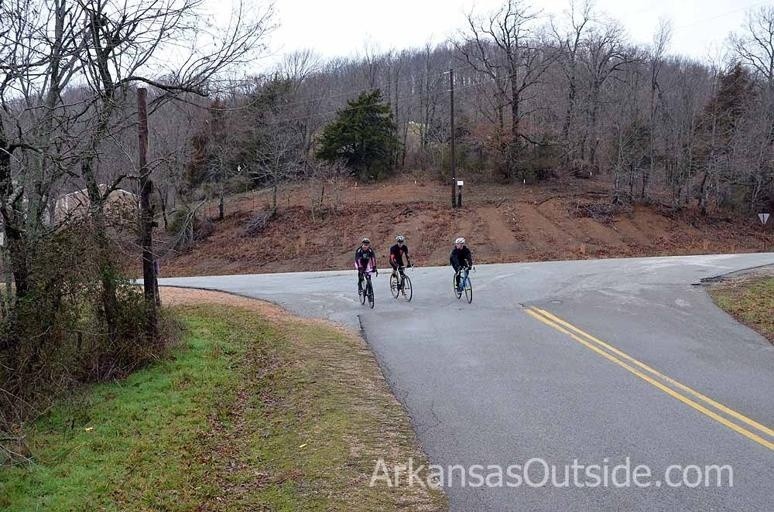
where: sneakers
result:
[457,287,462,293]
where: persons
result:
[388,236,412,291]
[355,238,376,303]
[450,238,473,291]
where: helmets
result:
[361,237,370,243]
[456,237,465,244]
[396,236,404,242]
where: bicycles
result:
[356,269,379,310]
[451,267,478,305]
[388,265,414,302]
